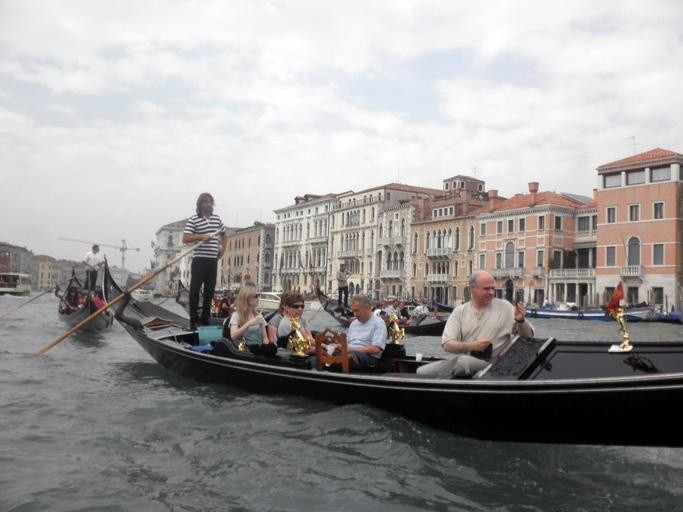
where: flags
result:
[604,281,623,317]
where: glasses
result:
[291,304,304,309]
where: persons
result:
[204,274,256,318]
[370,295,439,328]
[320,294,388,373]
[514,318,524,322]
[416,269,534,379]
[182,192,227,327]
[272,294,315,364]
[62,286,107,314]
[616,307,630,348]
[229,286,269,355]
[267,291,313,346]
[81,244,103,289]
[335,264,351,308]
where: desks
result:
[377,352,447,375]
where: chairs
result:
[348,342,406,373]
[264,304,309,367]
[212,311,264,362]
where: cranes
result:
[52,236,142,279]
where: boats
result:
[313,277,449,338]
[57,264,113,333]
[255,290,311,312]
[0,272,33,297]
[99,253,682,449]
[517,299,656,324]
[131,287,152,302]
[172,279,272,329]
